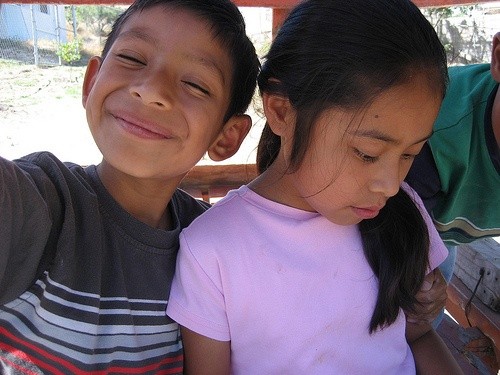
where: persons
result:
[165,0,466,375]
[0,1,241,374]
[404,31,500,330]
